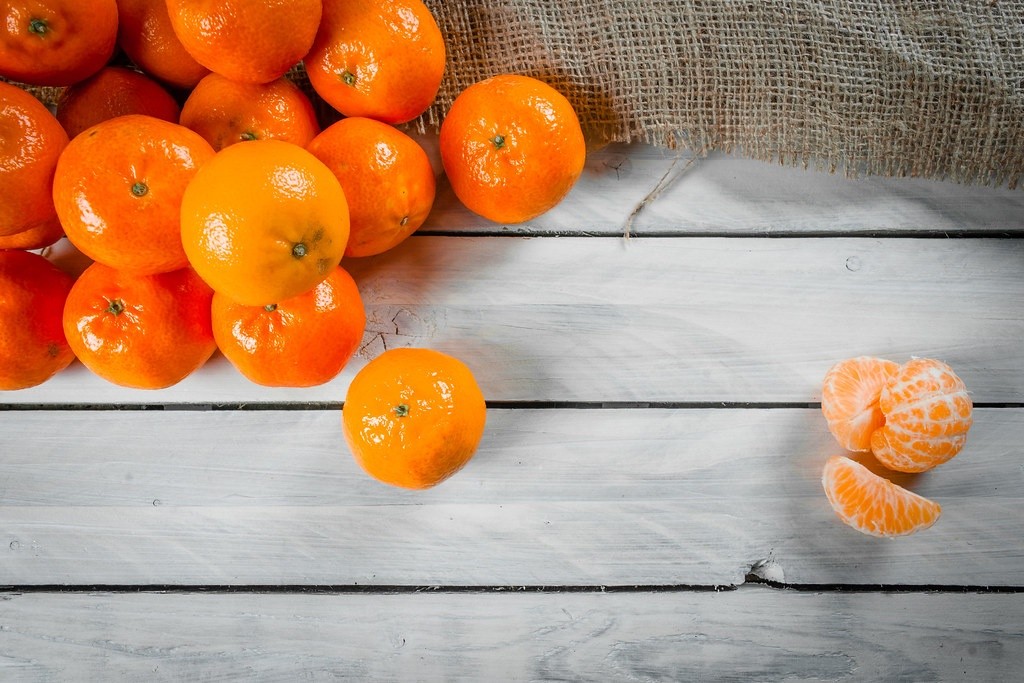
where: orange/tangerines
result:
[343,348,486,490]
[0,0,586,389]
[819,357,973,539]
